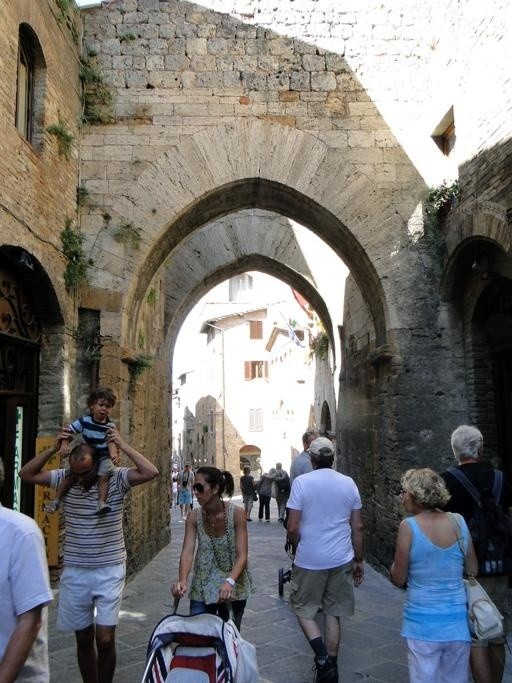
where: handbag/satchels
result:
[254,491,258,501]
[447,511,504,642]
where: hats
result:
[184,461,193,467]
[403,467,451,505]
[306,437,334,456]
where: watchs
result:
[226,577,236,587]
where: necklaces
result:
[205,501,233,572]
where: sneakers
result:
[95,503,112,516]
[44,499,61,514]
[314,655,338,683]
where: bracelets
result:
[352,557,364,563]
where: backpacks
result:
[445,466,510,578]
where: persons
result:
[240,466,256,521]
[286,436,365,683]
[171,467,255,638]
[43,387,120,515]
[261,463,289,523]
[0,457,54,683]
[18,423,160,683]
[440,424,512,683]
[390,467,479,683]
[176,464,193,521]
[289,432,318,490]
[256,468,275,523]
[171,465,178,509]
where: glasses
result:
[192,482,204,493]
[71,464,97,476]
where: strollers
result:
[140,597,257,682]
